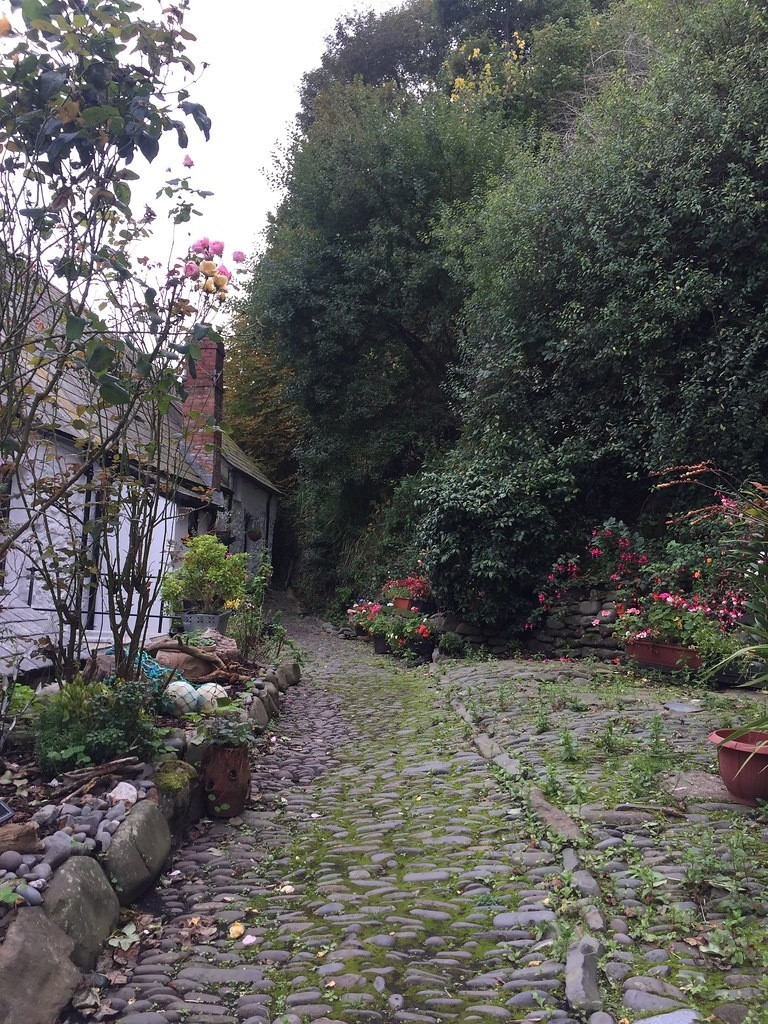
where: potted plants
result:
[159,534,253,636]
[181,702,261,818]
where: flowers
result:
[383,570,430,602]
[607,605,727,646]
[369,602,403,644]
[345,598,373,624]
[403,605,436,646]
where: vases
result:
[354,624,369,636]
[413,640,434,655]
[393,597,412,611]
[624,638,704,671]
[707,728,768,807]
[372,632,390,654]
